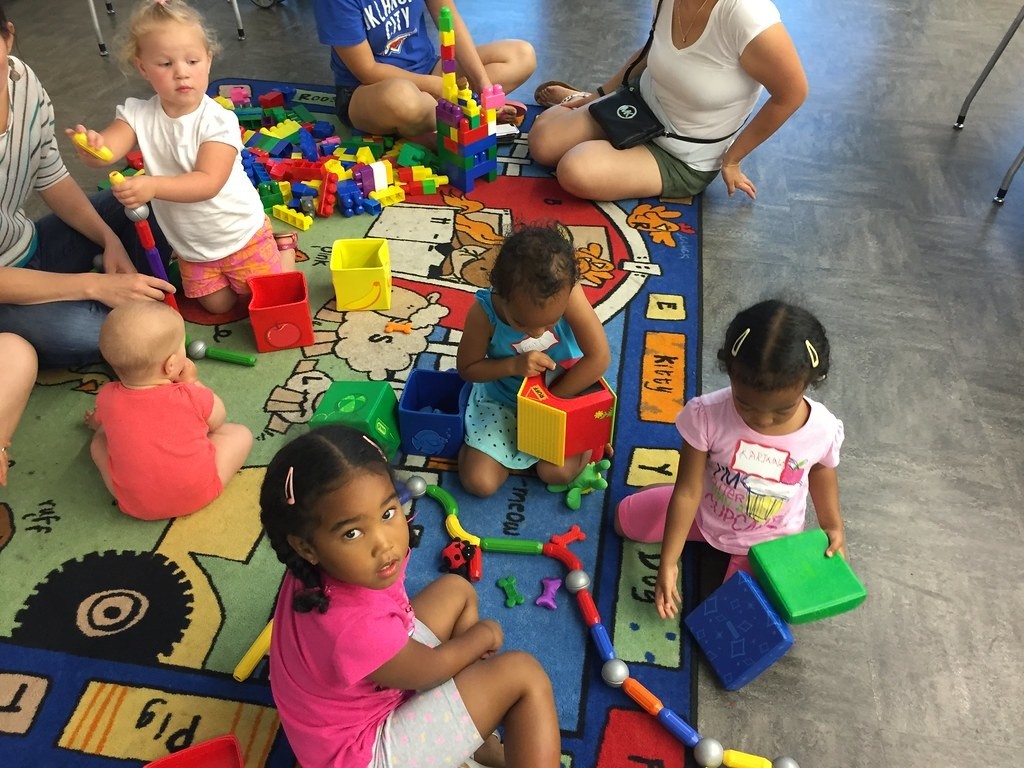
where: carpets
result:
[0,77,711,768]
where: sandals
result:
[272,229,300,250]
[496,101,529,124]
[534,78,595,108]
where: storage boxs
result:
[247,271,315,354]
[517,356,617,466]
[308,382,401,461]
[329,238,391,311]
[397,367,471,458]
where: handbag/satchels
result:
[588,83,669,148]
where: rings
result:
[2,447,7,453]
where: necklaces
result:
[677,0,708,42]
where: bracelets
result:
[596,87,605,97]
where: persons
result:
[614,299,846,619]
[84,301,253,521]
[0,332,39,487]
[456,226,611,498]
[528,0,808,202]
[65,0,299,314]
[311,0,536,140]
[0,8,176,376]
[259,425,560,768]
